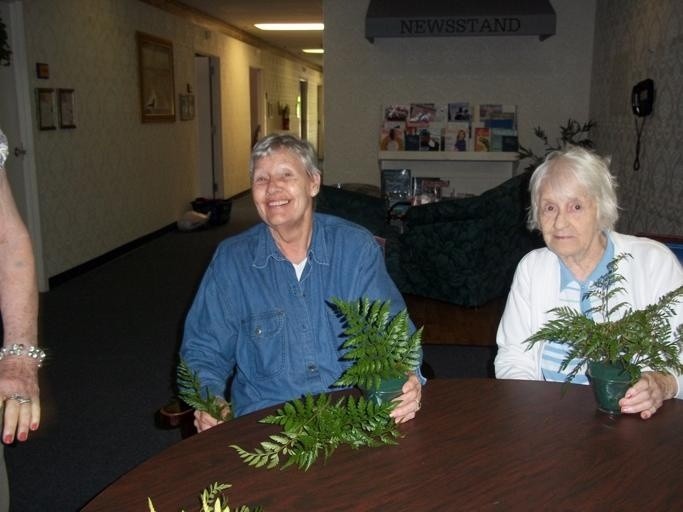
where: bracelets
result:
[0,341,49,369]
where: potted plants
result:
[524,248,683,419]
[326,297,420,416]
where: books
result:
[380,168,448,220]
[379,99,522,153]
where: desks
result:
[64,377,683,510]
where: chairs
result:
[402,173,537,311]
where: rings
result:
[413,398,423,412]
[8,392,24,401]
[18,397,33,405]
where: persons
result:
[176,132,428,434]
[493,144,682,420]
[0,129,44,511]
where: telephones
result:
[631,78,654,117]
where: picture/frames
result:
[136,30,177,125]
